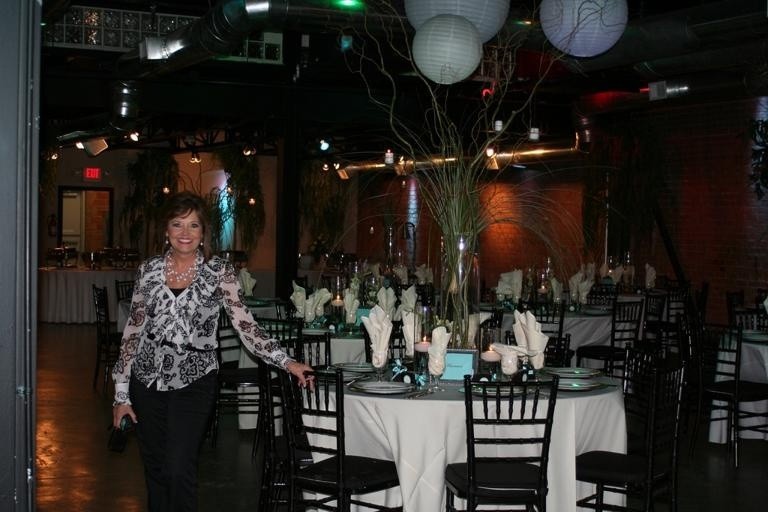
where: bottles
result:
[329,245,637,395]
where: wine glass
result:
[291,305,326,330]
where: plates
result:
[299,326,603,400]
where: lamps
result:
[45,131,258,163]
[323,144,499,171]
[405,0,629,84]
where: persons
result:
[111,189,315,511]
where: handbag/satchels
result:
[106,413,134,454]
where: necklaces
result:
[163,246,204,283]
